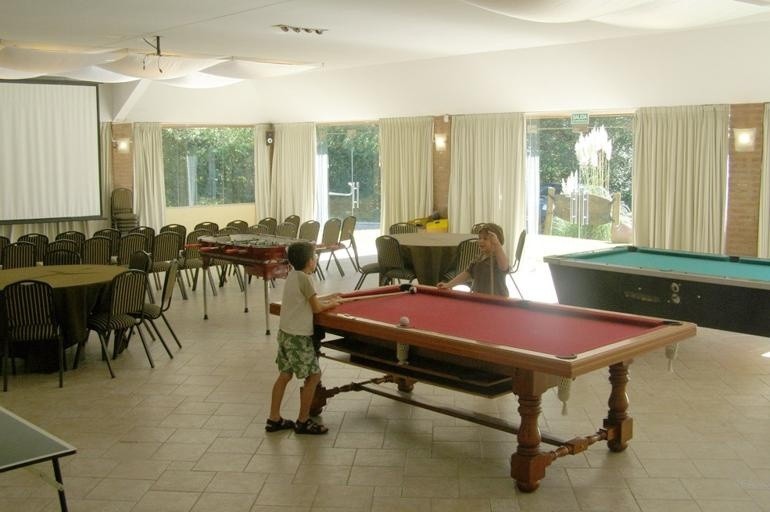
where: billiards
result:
[408,286,418,295]
[399,315,410,326]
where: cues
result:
[490,233,495,297]
[334,290,410,301]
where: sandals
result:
[295,419,328,434]
[266,417,293,431]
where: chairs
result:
[2,280,70,391]
[105,187,142,238]
[126,259,183,361]
[73,269,157,378]
[348,218,528,301]
[122,249,153,349]
[1,213,361,296]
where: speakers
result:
[266,132,273,145]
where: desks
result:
[195,232,315,336]
[541,244,770,374]
[268,284,698,495]
[0,403,78,511]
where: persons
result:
[265,241,343,435]
[436,222,510,298]
